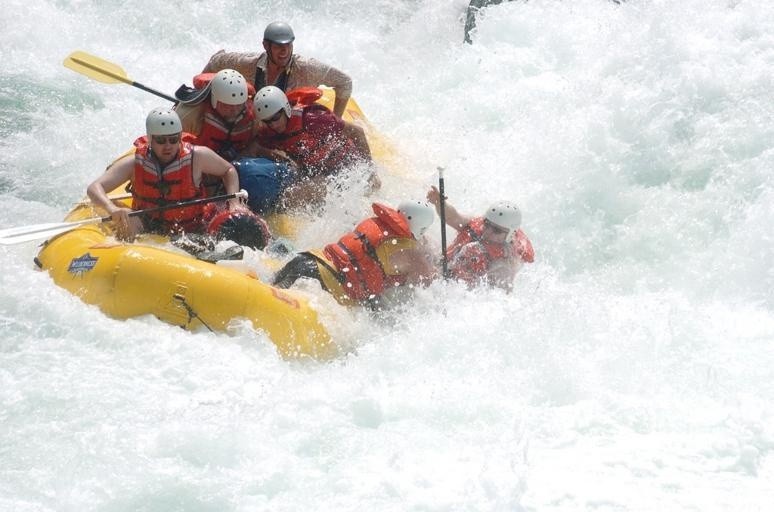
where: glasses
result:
[153,135,178,144]
[484,221,506,234]
[262,109,283,124]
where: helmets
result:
[397,196,435,240]
[146,106,182,141]
[264,21,295,44]
[211,69,248,108]
[484,200,521,243]
[254,86,291,123]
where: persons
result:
[263,186,535,304]
[87,21,381,262]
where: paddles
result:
[0,188,248,245]
[62,50,178,104]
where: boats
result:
[32,89,404,368]
